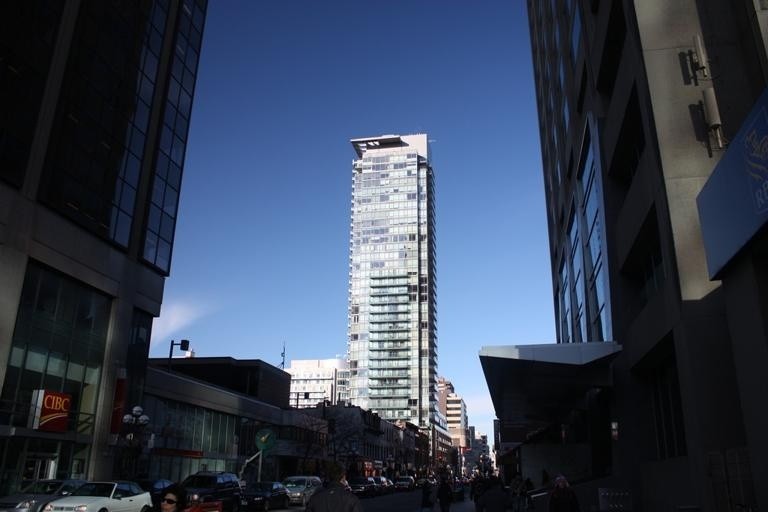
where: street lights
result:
[122,406,150,484]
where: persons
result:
[470,466,580,511]
[435,474,456,512]
[305,462,362,512]
[421,481,435,512]
[160,485,188,512]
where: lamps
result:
[688,33,732,159]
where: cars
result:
[0,479,92,512]
[43,479,154,512]
[181,471,243,512]
[351,476,434,498]
[284,475,324,506]
[242,481,291,510]
